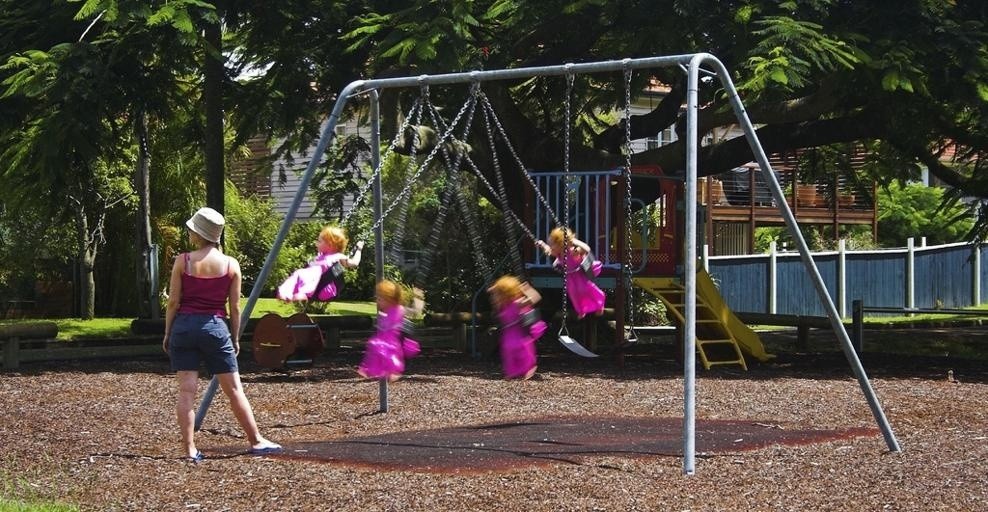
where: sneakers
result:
[251,439,282,452]
[185,450,210,464]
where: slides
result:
[697,268,767,362]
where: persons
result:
[161,207,281,461]
[531,226,606,322]
[486,275,549,382]
[354,279,423,384]
[275,227,365,305]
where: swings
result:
[558,70,640,357]
[307,85,594,346]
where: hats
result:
[186,207,225,244]
